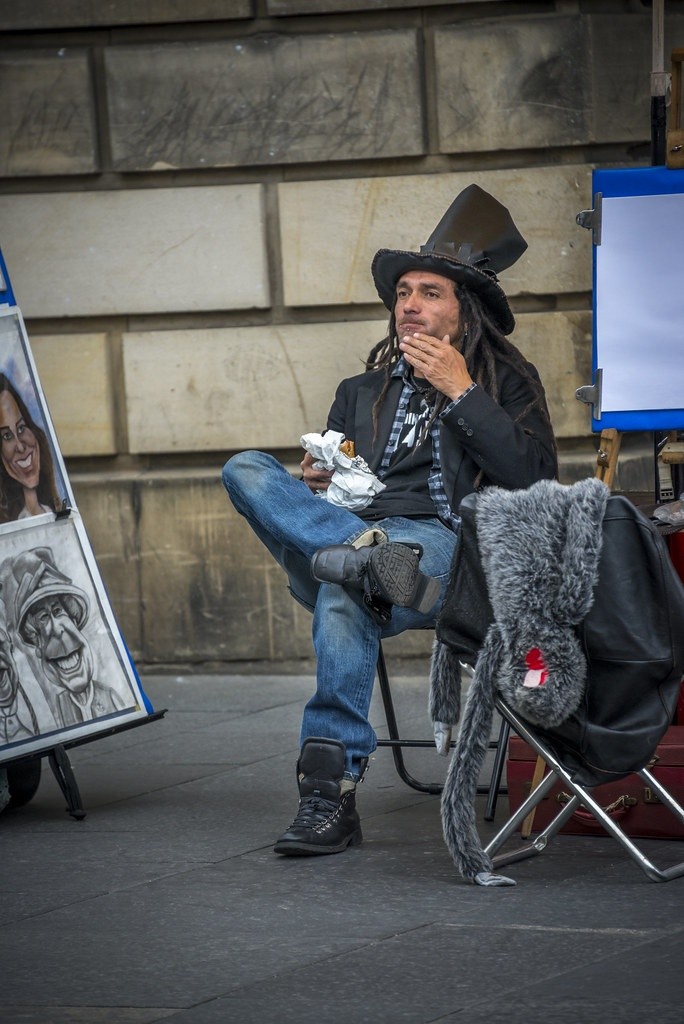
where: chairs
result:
[365,493,684,882]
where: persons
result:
[221,185,561,856]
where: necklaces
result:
[410,371,434,394]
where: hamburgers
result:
[340,439,354,460]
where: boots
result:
[311,541,443,614]
[272,738,369,853]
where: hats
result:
[371,183,527,337]
